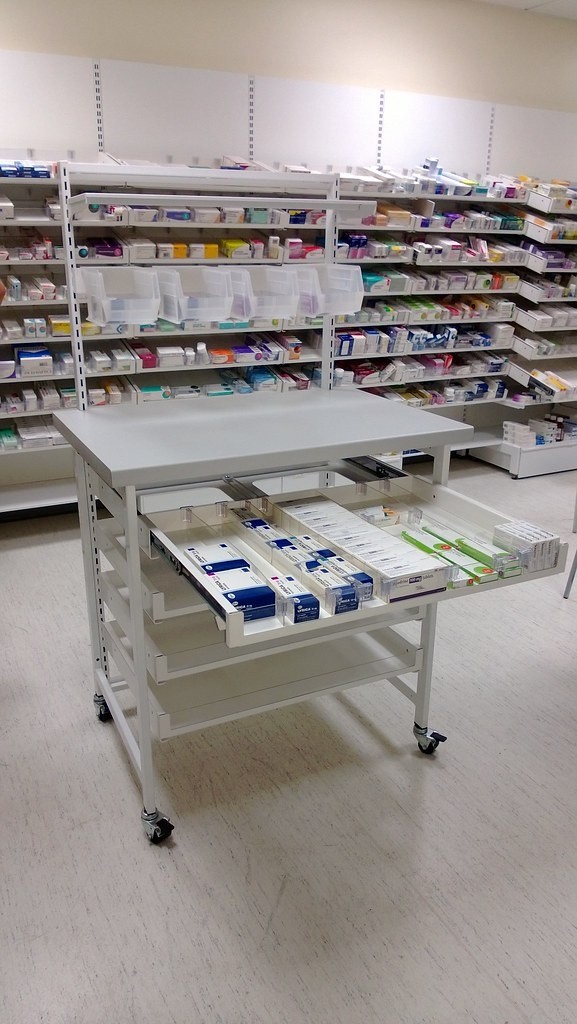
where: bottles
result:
[544,413,565,441]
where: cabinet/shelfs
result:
[466,190,577,481]
[0,176,529,514]
[50,162,573,847]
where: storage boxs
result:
[0,157,577,626]
